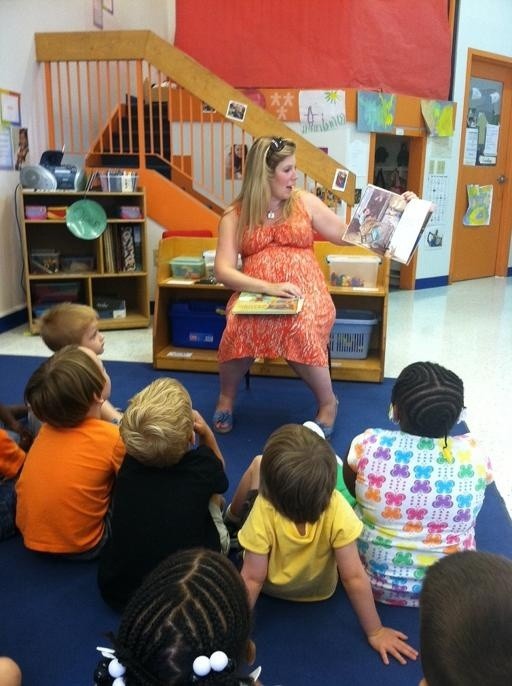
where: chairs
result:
[162,229,213,240]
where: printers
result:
[39,150,80,192]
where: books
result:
[230,290,305,314]
[340,182,436,267]
[103,223,137,274]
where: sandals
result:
[211,405,234,434]
[313,395,340,441]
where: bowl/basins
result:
[99,175,138,192]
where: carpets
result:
[1,352,512,684]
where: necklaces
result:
[261,201,281,220]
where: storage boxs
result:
[166,299,230,351]
[31,302,74,324]
[324,254,382,288]
[203,249,243,282]
[31,280,82,304]
[28,250,63,274]
[166,255,205,280]
[62,254,96,270]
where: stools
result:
[225,291,337,394]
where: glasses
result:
[266,136,286,159]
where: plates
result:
[64,198,108,240]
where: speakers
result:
[20,163,57,190]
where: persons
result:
[40,301,127,424]
[95,376,231,614]
[13,128,30,171]
[94,544,265,684]
[224,422,419,667]
[342,362,494,610]
[0,654,23,685]
[211,134,421,443]
[14,342,129,566]
[1,404,33,547]
[418,550,511,685]
[226,146,248,176]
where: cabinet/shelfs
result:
[21,187,150,333]
[150,236,392,384]
